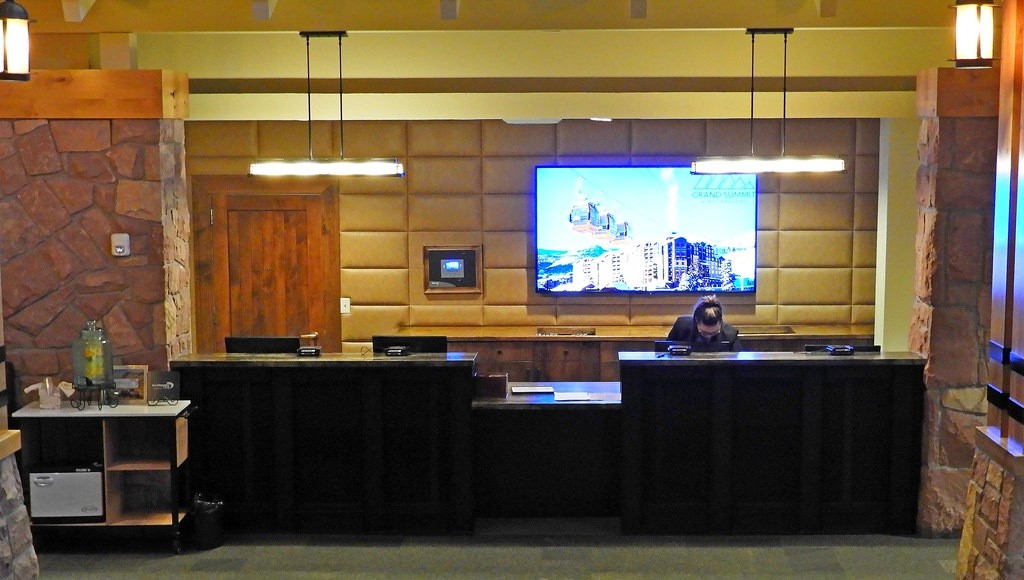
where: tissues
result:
[24,381,76,409]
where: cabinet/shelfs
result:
[11,400,199,555]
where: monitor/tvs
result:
[534,164,758,293]
[372,335,448,354]
[224,337,301,354]
[805,345,881,353]
[655,340,730,353]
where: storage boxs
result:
[28,472,104,524]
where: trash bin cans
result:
[190,492,229,550]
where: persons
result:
[666,294,743,352]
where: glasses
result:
[697,326,721,335]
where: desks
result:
[619,352,929,537]
[168,351,480,538]
[471,382,623,519]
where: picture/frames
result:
[101,364,149,406]
[422,245,484,295]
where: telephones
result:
[827,344,855,355]
[297,346,321,357]
[384,346,411,355]
[668,345,692,355]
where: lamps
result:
[0,0,40,82]
[689,28,846,175]
[247,31,404,178]
[946,0,1002,70]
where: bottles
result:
[71,328,112,388]
